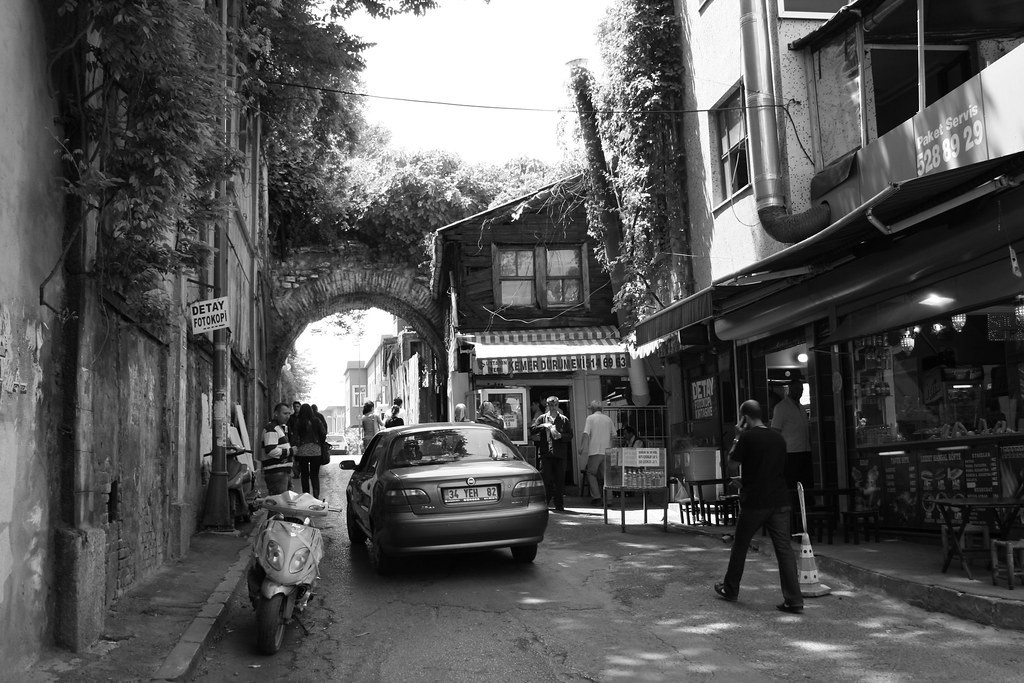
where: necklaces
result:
[757,425,766,427]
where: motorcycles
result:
[247,489,344,656]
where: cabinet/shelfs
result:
[603,447,668,533]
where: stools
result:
[719,496,739,518]
[790,511,836,545]
[704,500,728,526]
[990,536,1024,590]
[677,497,699,525]
[941,525,990,564]
[841,510,880,546]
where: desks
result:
[804,487,863,545]
[685,478,739,527]
[923,497,1024,580]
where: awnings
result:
[631,265,811,394]
[454,325,634,377]
[711,151,1024,293]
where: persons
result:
[714,400,804,613]
[451,393,563,465]
[361,396,408,456]
[620,426,646,449]
[529,396,573,512]
[286,401,327,499]
[770,380,815,534]
[260,403,298,520]
[579,400,617,506]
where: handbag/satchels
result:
[321,441,331,465]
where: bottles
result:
[623,469,665,488]
[854,333,896,444]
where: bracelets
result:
[732,439,740,445]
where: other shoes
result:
[592,498,612,507]
[293,473,299,479]
[554,505,564,512]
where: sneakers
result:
[714,581,739,603]
[777,601,804,612]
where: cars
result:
[324,434,349,454]
[338,421,550,572]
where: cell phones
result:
[740,418,746,428]
